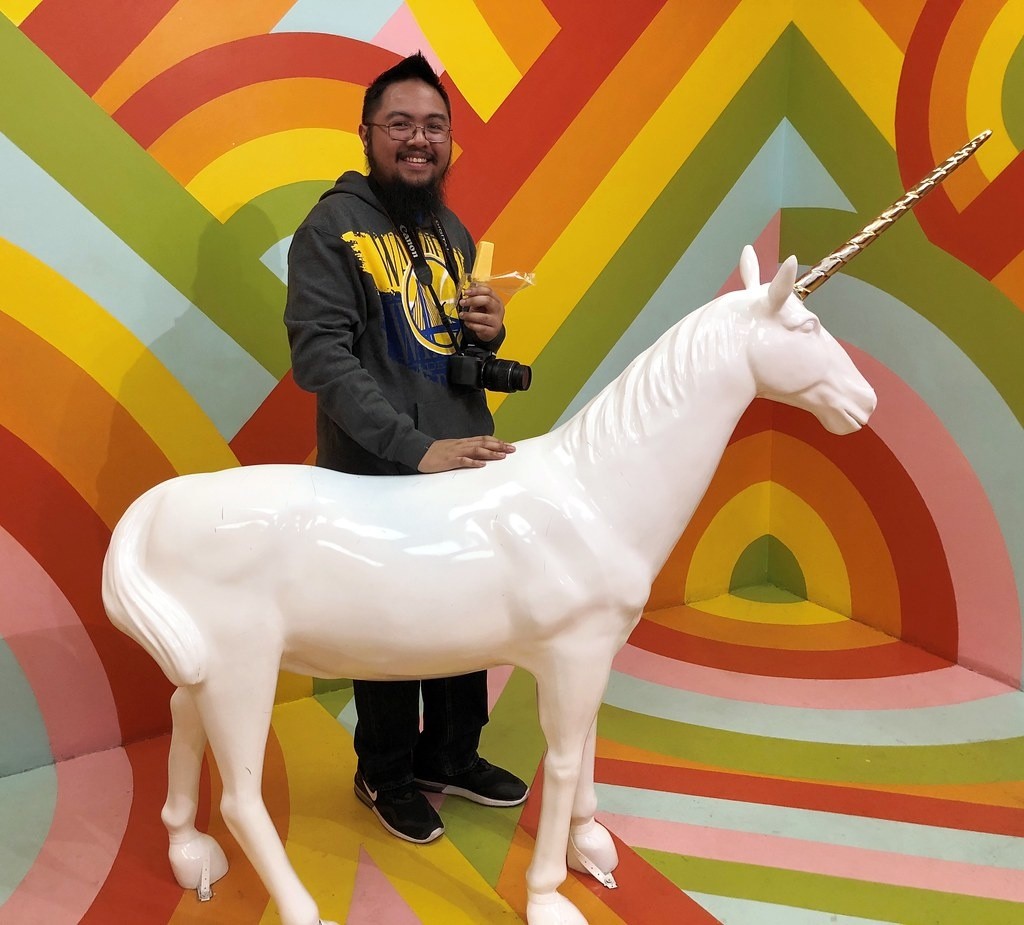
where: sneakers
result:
[411,751,531,807]
[354,769,444,844]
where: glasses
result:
[362,119,453,143]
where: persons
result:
[283,49,531,845]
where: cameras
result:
[450,346,532,393]
